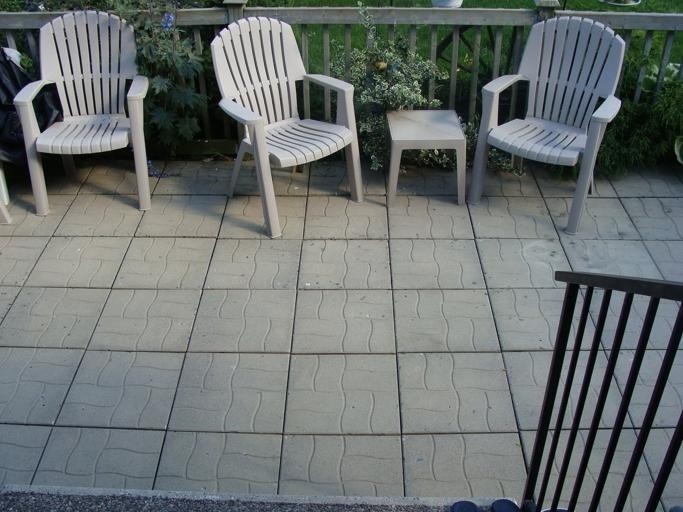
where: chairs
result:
[464,15,626,236]
[12,10,151,217]
[209,16,364,239]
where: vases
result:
[429,1,464,10]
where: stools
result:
[383,109,467,209]
